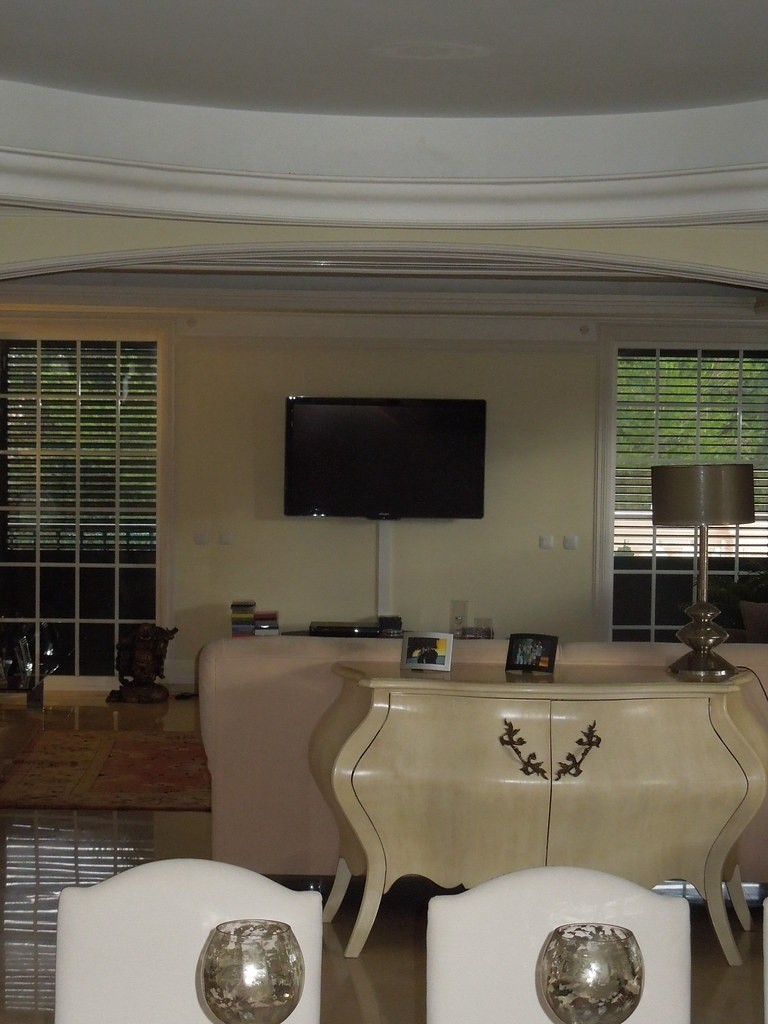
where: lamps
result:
[652,464,755,682]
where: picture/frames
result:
[400,632,453,672]
[504,633,558,674]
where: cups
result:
[450,600,468,638]
[474,618,492,635]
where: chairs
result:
[425,865,691,1024]
[54,857,322,1024]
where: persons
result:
[516,640,543,666]
[417,646,438,664]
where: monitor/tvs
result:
[284,396,486,518]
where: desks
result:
[308,660,768,966]
